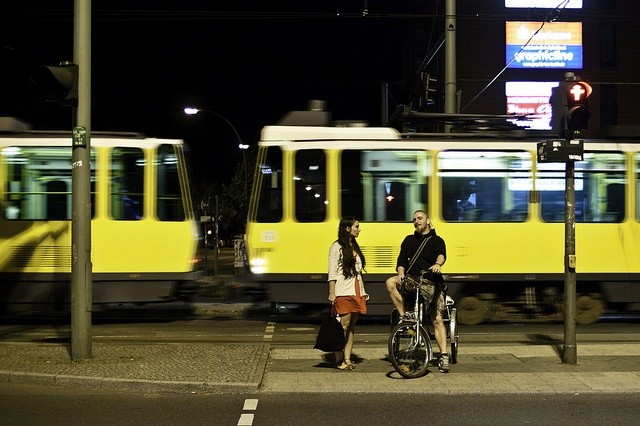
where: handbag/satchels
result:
[313,317,345,352]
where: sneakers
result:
[396,316,409,331]
[438,353,449,372]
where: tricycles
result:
[389,270,459,378]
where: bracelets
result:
[436,263,442,266]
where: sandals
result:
[333,360,352,369]
[344,359,356,369]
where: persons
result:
[328,217,369,369]
[386,211,449,372]
[457,193,475,220]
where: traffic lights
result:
[563,71,599,132]
[419,70,440,110]
[40,61,82,105]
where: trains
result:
[0,100,640,326]
